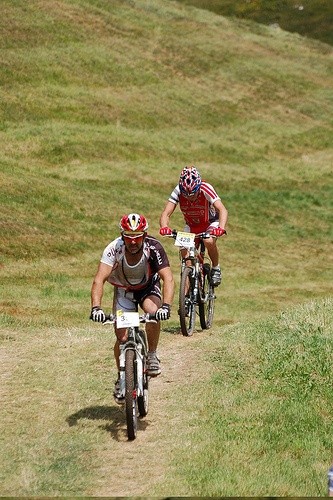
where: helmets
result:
[120,213,149,237]
[179,166,201,198]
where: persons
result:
[159,168,228,314]
[90,213,174,404]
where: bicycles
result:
[162,229,228,337]
[89,302,170,441]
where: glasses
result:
[123,234,143,243]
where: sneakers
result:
[210,264,222,286]
[178,297,189,314]
[114,379,126,405]
[145,352,162,376]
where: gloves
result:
[155,303,170,320]
[212,228,227,237]
[160,226,172,236]
[90,306,106,322]
